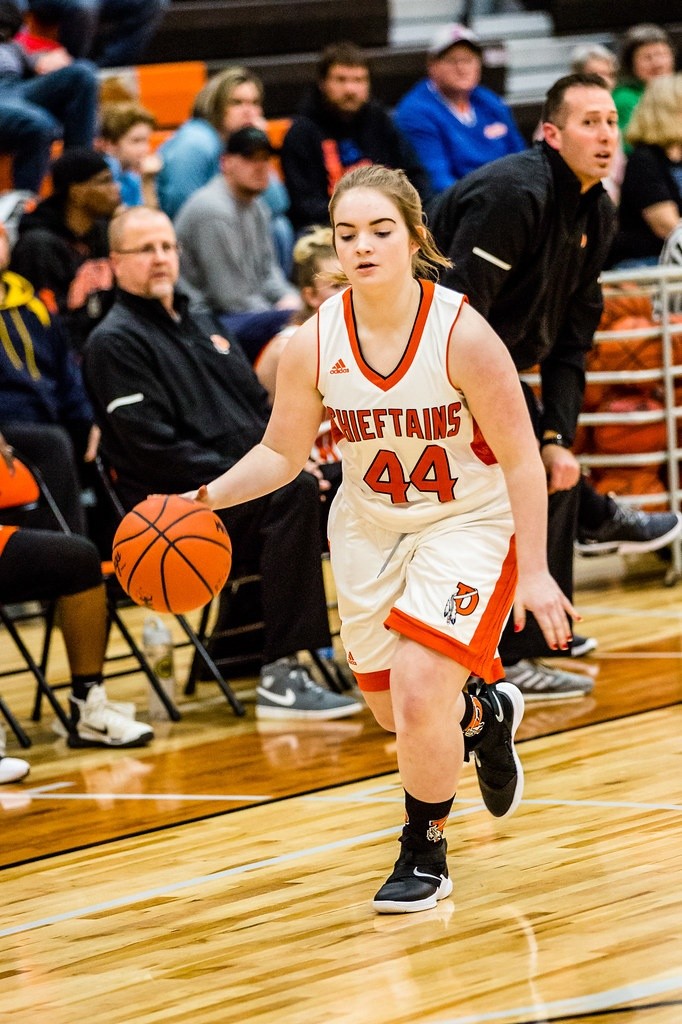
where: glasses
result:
[112,239,180,255]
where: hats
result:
[428,22,484,55]
[50,149,112,183]
[225,127,270,156]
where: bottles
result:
[141,614,175,716]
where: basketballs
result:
[111,495,233,614]
[519,281,682,513]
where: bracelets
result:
[541,439,571,449]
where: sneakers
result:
[67,682,155,749]
[0,754,31,784]
[503,661,593,700]
[574,491,682,560]
[255,659,362,719]
[570,635,597,657]
[372,826,453,912]
[467,682,525,821]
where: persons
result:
[148,164,584,915]
[0,0,682,788]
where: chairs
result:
[0,447,181,747]
[95,444,343,716]
